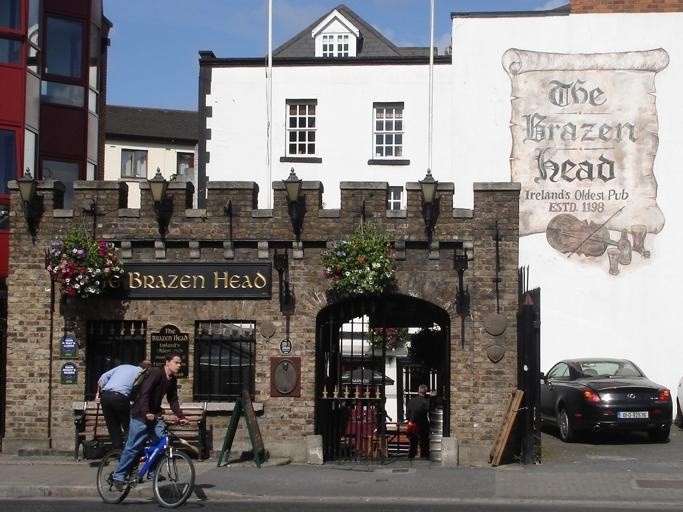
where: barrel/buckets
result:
[428,435,444,464]
[429,409,444,435]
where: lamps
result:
[16,167,42,223]
[281,166,304,225]
[418,167,438,229]
[146,166,170,223]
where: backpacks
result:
[132,366,163,390]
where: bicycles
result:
[95,416,195,508]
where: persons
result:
[374,396,392,434]
[406,384,431,458]
[108,351,189,488]
[94,360,152,461]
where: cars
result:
[540,358,672,440]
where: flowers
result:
[368,328,408,353]
[43,222,127,300]
[319,225,400,302]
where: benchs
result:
[73,402,207,463]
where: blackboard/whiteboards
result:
[489,389,523,458]
[241,387,267,454]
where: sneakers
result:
[147,471,166,482]
[107,473,124,492]
